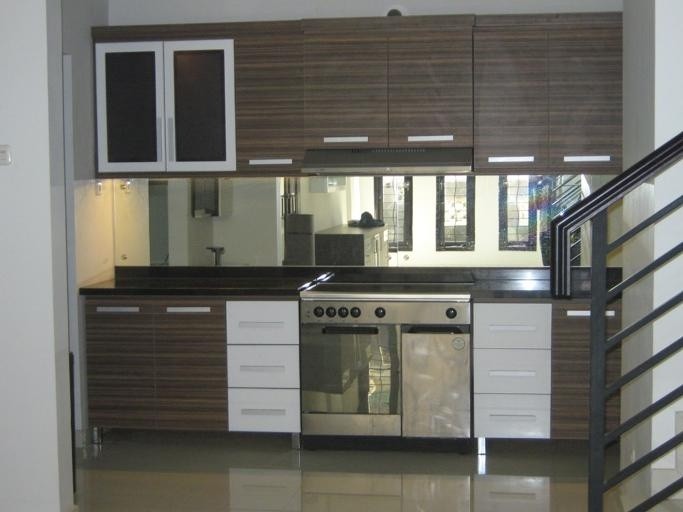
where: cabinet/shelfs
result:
[80,276,320,450]
[314,221,390,266]
[89,19,300,178]
[472,474,550,512]
[472,288,621,455]
[228,454,301,511]
[300,13,474,150]
[473,11,622,176]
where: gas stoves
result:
[300,283,470,325]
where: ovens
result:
[300,321,403,415]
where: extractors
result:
[299,148,475,176]
